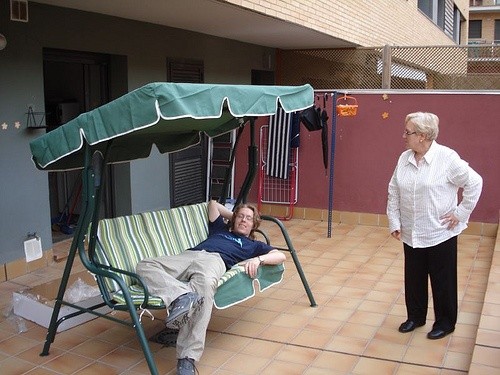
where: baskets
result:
[336,96,358,117]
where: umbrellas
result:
[321,93,329,176]
[300,95,322,132]
[290,112,300,147]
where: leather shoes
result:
[428,326,454,340]
[399,319,426,333]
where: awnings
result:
[29,84,314,172]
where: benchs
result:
[87,201,284,311]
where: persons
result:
[135,200,286,375]
[386,112,483,339]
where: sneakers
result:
[177,359,199,375]
[164,292,205,329]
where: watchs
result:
[258,256,264,265]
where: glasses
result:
[404,129,416,136]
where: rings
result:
[449,218,452,220]
